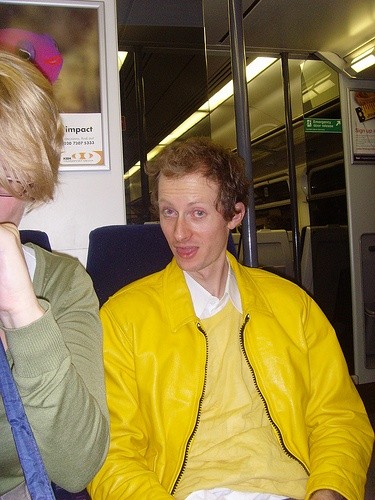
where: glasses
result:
[0,176,35,199]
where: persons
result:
[0,50,111,500]
[85,139,375,500]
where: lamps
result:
[350,46,375,74]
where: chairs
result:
[18,225,356,376]
